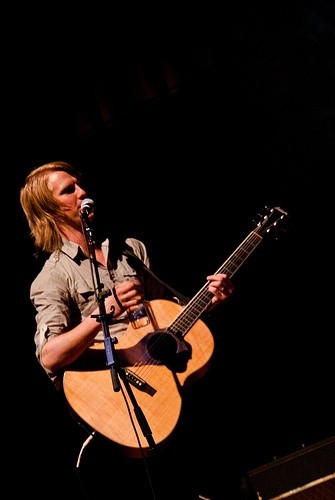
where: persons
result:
[20,162,235,384]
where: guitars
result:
[63,202,289,459]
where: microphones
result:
[78,198,94,216]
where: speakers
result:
[243,437,334,498]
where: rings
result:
[219,287,226,291]
